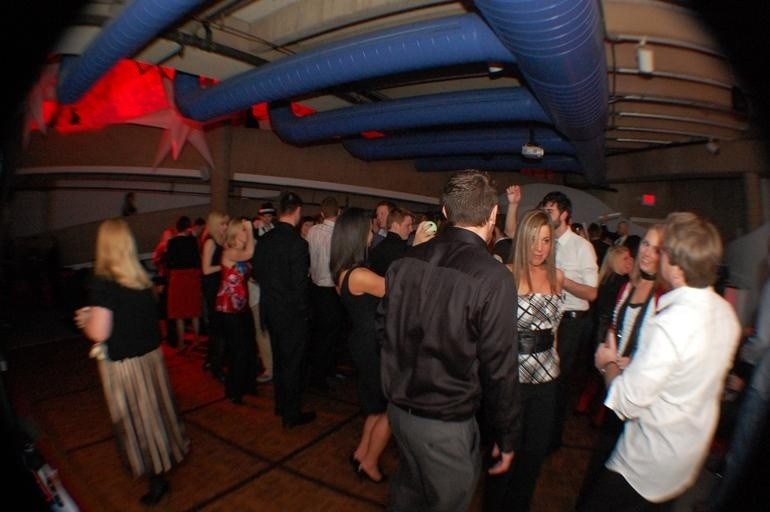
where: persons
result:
[153,169,769,512]
[71,216,195,509]
[120,190,138,216]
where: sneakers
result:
[274,404,317,430]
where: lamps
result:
[636,36,655,76]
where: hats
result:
[257,203,277,216]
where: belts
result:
[394,403,473,422]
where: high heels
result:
[349,453,390,484]
[139,481,170,505]
